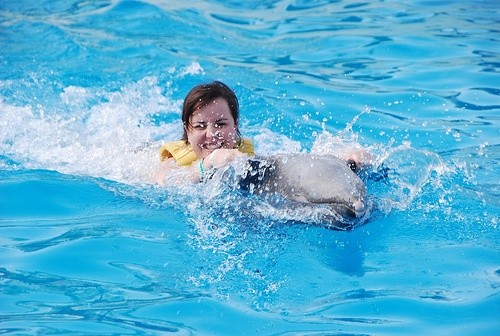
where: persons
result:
[160,79,255,185]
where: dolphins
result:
[255,154,366,231]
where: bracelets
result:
[197,157,207,180]
